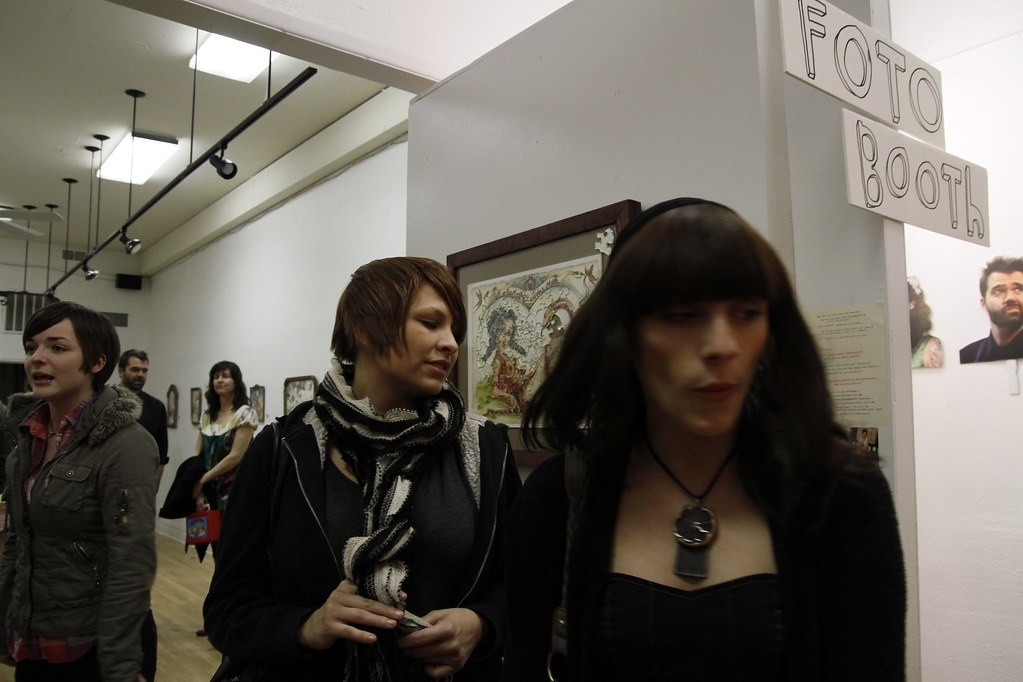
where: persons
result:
[480,196,906,682]
[201,256,523,682]
[185,360,258,651]
[959,256,1023,365]
[907,275,943,368]
[112,349,169,497]
[0,301,160,681]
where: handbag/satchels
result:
[186,503,222,544]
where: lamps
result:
[80,265,101,282]
[209,154,238,180]
[96,130,180,186]
[119,235,142,255]
[188,32,281,85]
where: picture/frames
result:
[248,384,265,425]
[165,383,179,430]
[283,376,319,417]
[905,253,1023,369]
[190,387,202,426]
[441,199,644,475]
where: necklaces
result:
[640,424,740,583]
